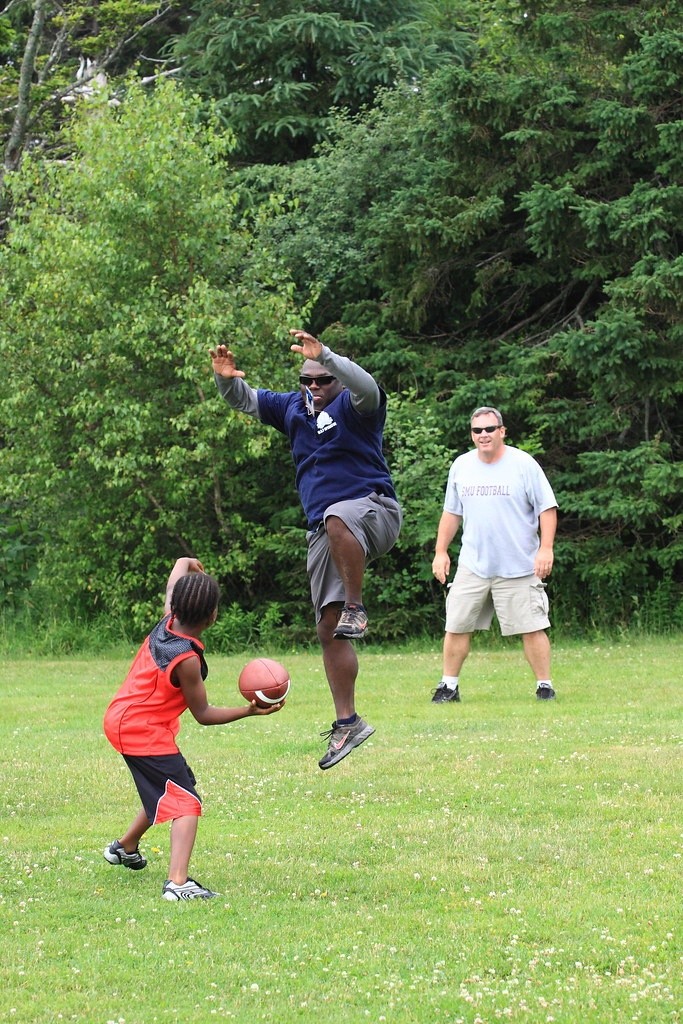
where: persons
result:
[208,327,403,770]
[101,556,286,903]
[430,407,558,705]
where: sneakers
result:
[330,602,371,639]
[432,681,461,704]
[315,712,375,768]
[102,840,147,870]
[535,683,556,702]
[160,878,224,903]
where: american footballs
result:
[238,658,291,709]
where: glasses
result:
[297,373,337,387]
[471,425,503,434]
